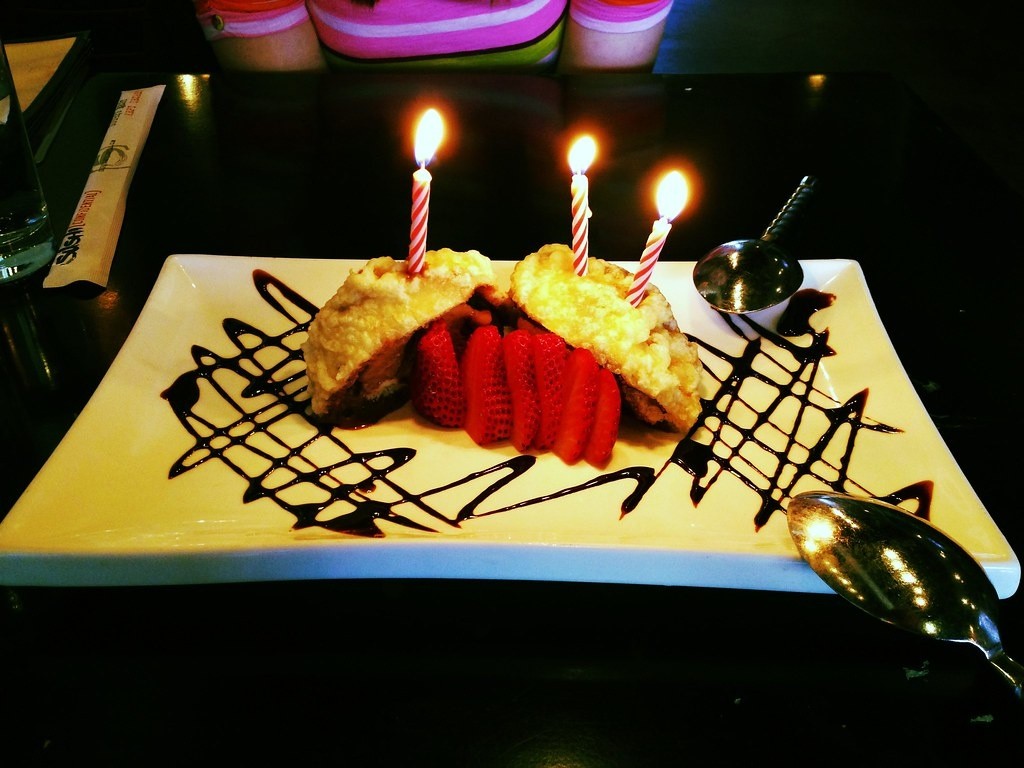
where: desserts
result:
[508,244,704,435]
[302,246,498,417]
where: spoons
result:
[787,490,1024,718]
[693,169,821,315]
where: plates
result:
[0,250,1020,614]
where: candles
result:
[406,107,446,275]
[564,133,599,277]
[626,168,693,308]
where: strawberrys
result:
[408,326,621,467]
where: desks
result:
[0,72,1024,768]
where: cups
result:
[0,38,55,287]
